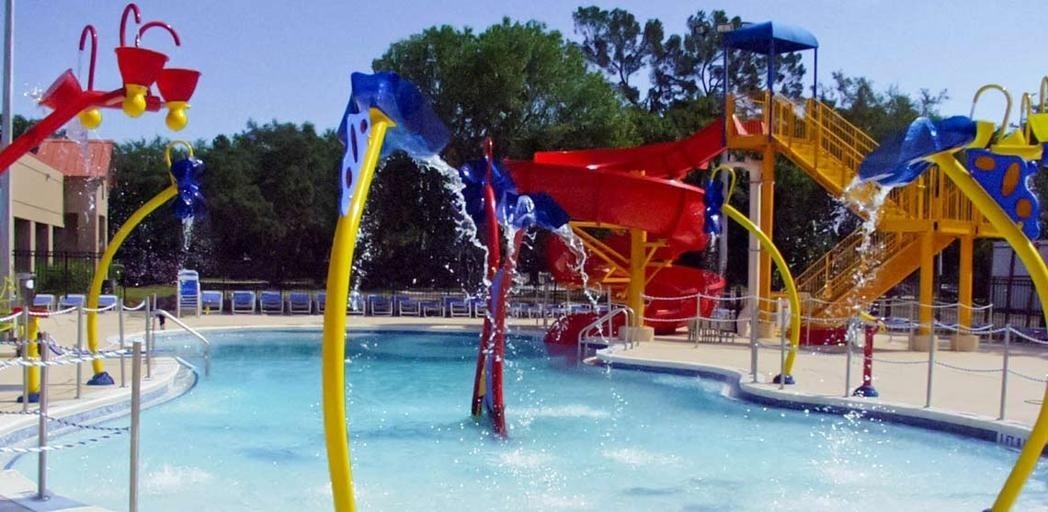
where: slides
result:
[504,116,769,344]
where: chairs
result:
[31,292,121,312]
[971,321,1048,346]
